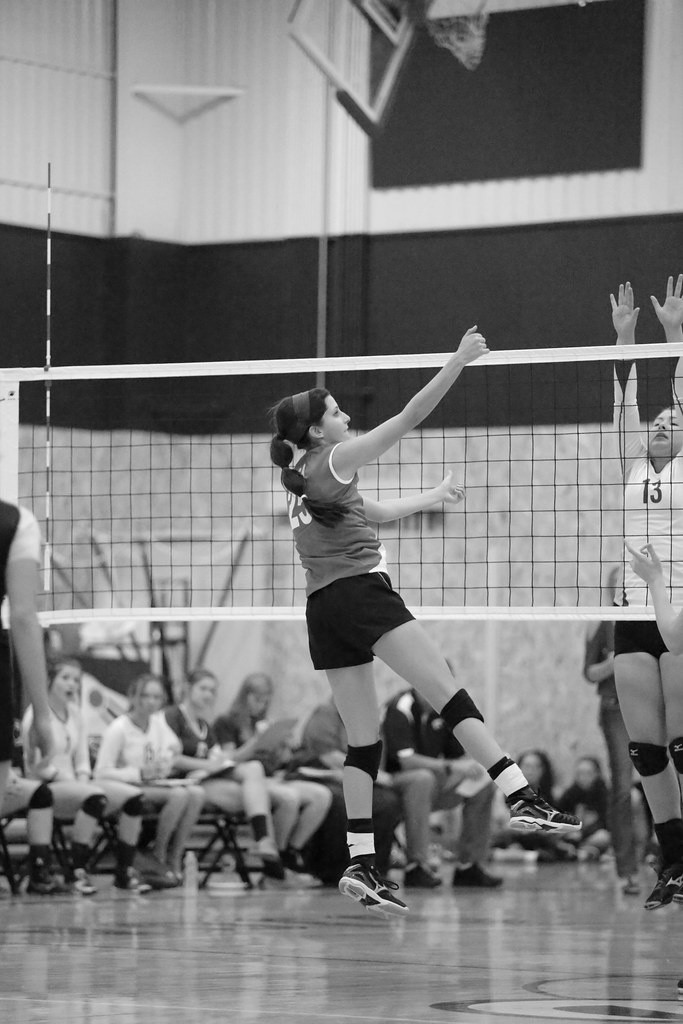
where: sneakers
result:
[49,866,70,893]
[338,859,410,922]
[110,866,153,895]
[68,868,97,895]
[28,867,59,894]
[643,840,662,873]
[248,836,280,864]
[514,836,593,864]
[506,786,584,834]
[644,860,683,910]
[262,869,323,895]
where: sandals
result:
[619,872,641,895]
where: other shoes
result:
[598,845,617,863]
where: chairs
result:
[0,808,260,896]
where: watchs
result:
[445,759,453,776]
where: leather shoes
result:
[452,862,501,886]
[404,865,441,887]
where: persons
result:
[0,499,58,813]
[271,326,583,919]
[610,273,683,911]
[93,673,205,888]
[584,563,662,896]
[377,657,504,888]
[21,657,152,896]
[622,539,683,655]
[1,656,657,897]
